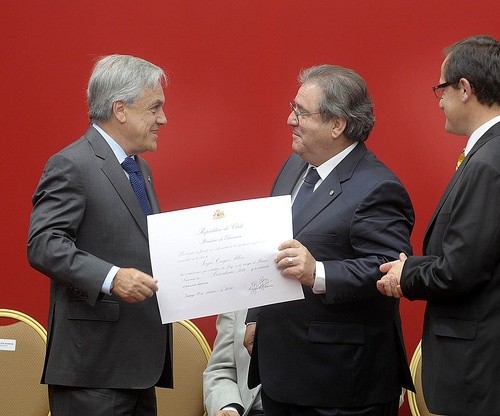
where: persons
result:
[203,309,264,416]
[243,64,416,415]
[28,54,177,416]
[377,36,500,415]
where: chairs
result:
[155,319,212,416]
[0,309,51,416]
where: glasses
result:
[289,101,322,122]
[432,78,474,99]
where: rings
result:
[289,257,293,267]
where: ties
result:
[456,151,466,171]
[121,157,153,220]
[292,167,321,221]
